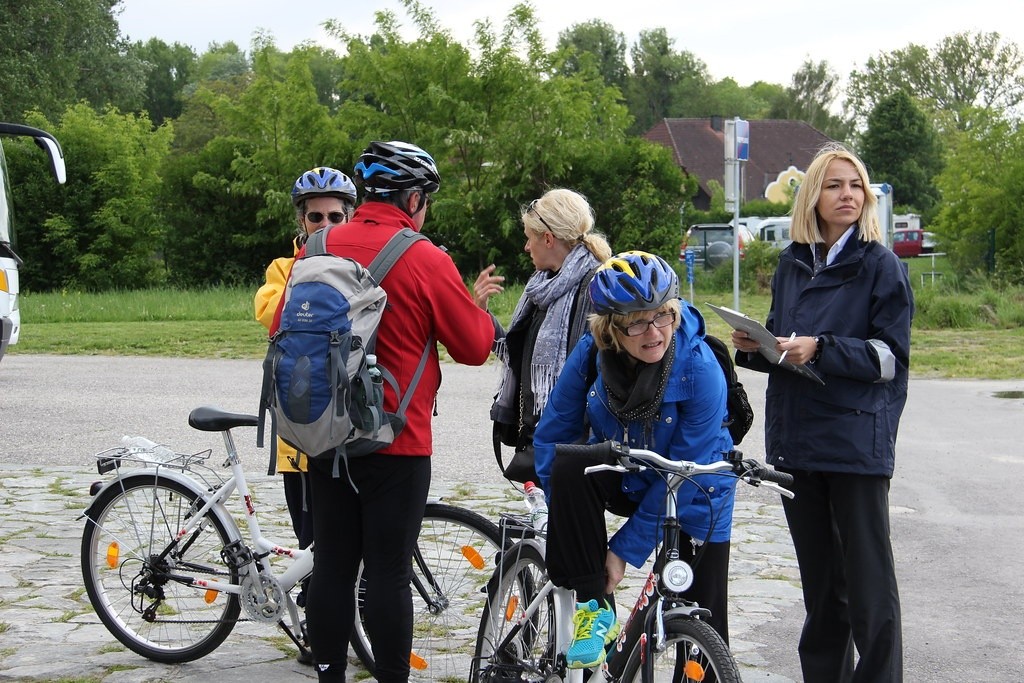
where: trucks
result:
[870,184,893,254]
[730,218,793,253]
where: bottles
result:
[525,481,548,542]
[357,354,382,408]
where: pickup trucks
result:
[893,229,948,258]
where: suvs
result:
[679,225,756,269]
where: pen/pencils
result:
[777,331,796,365]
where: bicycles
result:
[470,442,794,683]
[74,403,538,683]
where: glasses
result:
[525,199,558,239]
[305,210,347,225]
[610,312,678,338]
[419,193,433,208]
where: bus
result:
[0,120,67,359]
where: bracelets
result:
[809,336,819,364]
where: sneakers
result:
[565,597,618,670]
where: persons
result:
[532,250,739,683]
[732,142,914,683]
[473,189,612,483]
[255,141,495,683]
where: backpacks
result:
[264,225,432,461]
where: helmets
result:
[292,167,357,206]
[355,140,441,193]
[587,250,683,316]
